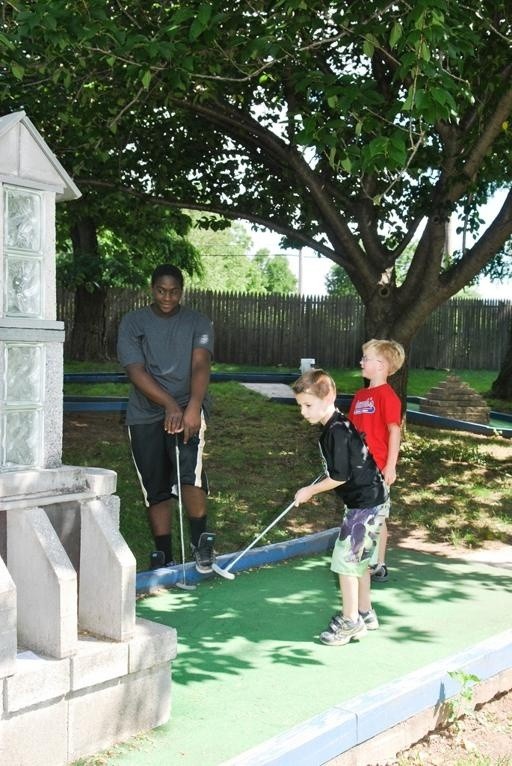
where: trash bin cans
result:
[300,358,315,375]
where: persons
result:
[342,336,408,583]
[115,262,218,575]
[290,366,393,645]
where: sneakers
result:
[320,606,381,646]
[188,532,218,574]
[370,562,390,583]
[147,551,176,573]
